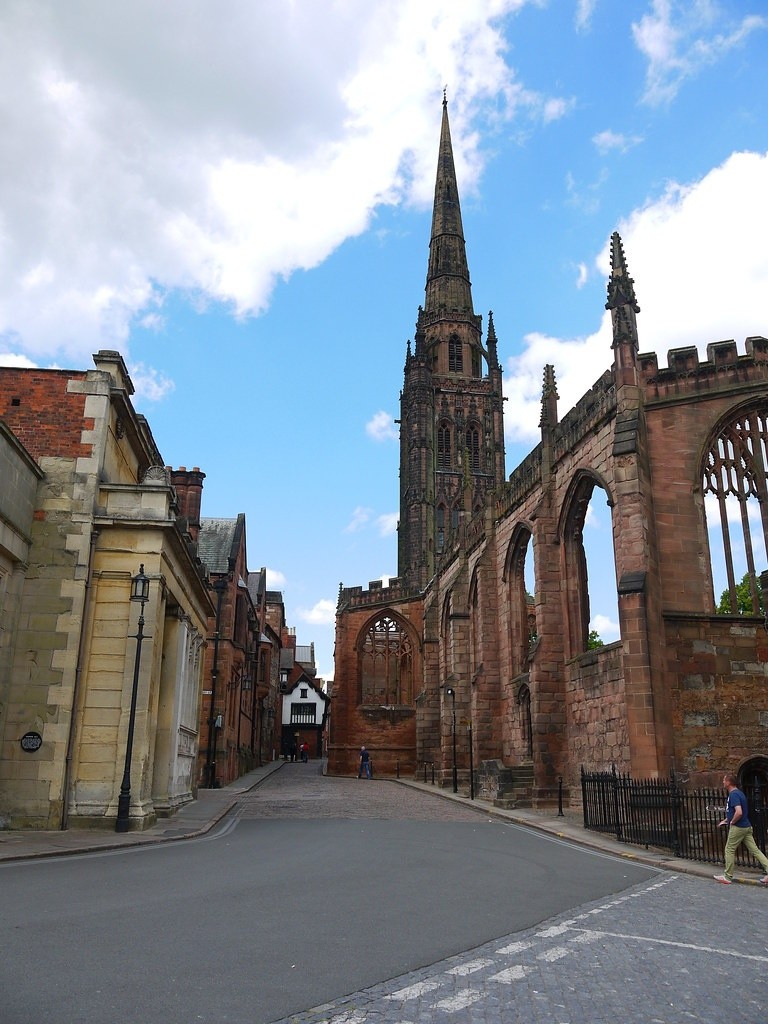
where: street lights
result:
[448,687,458,791]
[114,562,152,833]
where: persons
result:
[356,746,370,779]
[300,741,310,763]
[714,773,768,884]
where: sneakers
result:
[713,875,731,884]
[760,875,768,883]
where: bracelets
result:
[731,821,732,824]
